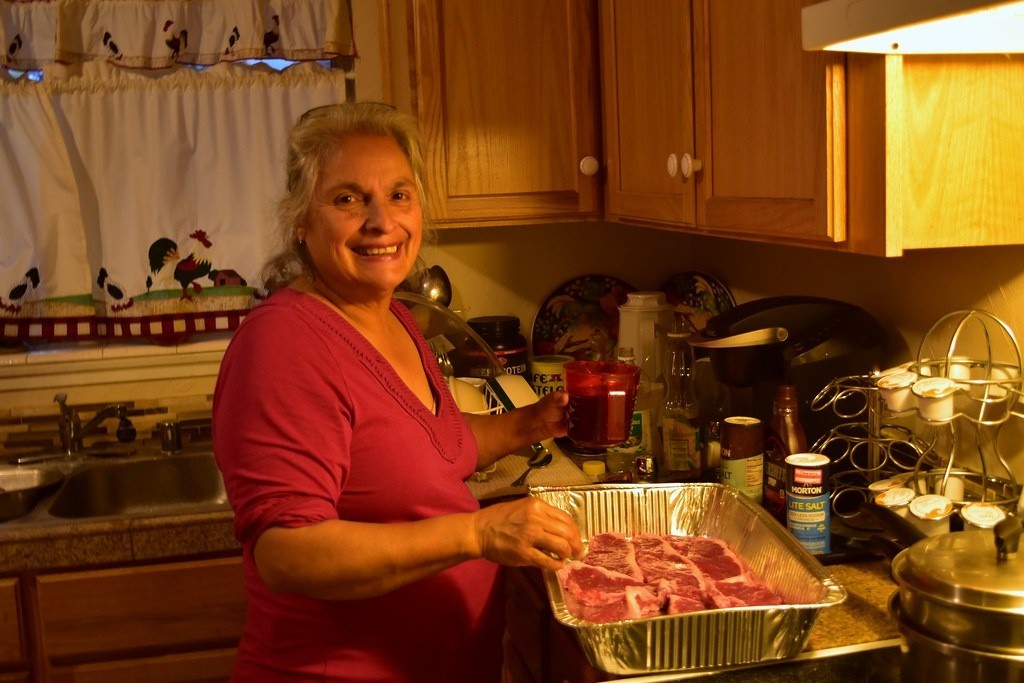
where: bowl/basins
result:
[448,375,490,415]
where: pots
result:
[886,512,1024,683]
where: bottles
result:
[605,347,658,475]
[662,320,704,480]
[761,383,809,528]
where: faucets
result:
[53,391,126,453]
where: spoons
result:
[511,448,553,486]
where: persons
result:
[210,102,582,683]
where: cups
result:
[563,360,641,447]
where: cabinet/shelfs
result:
[596,0,1024,259]
[0,554,247,683]
[501,565,614,683]
[378,0,601,234]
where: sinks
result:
[47,453,221,518]
